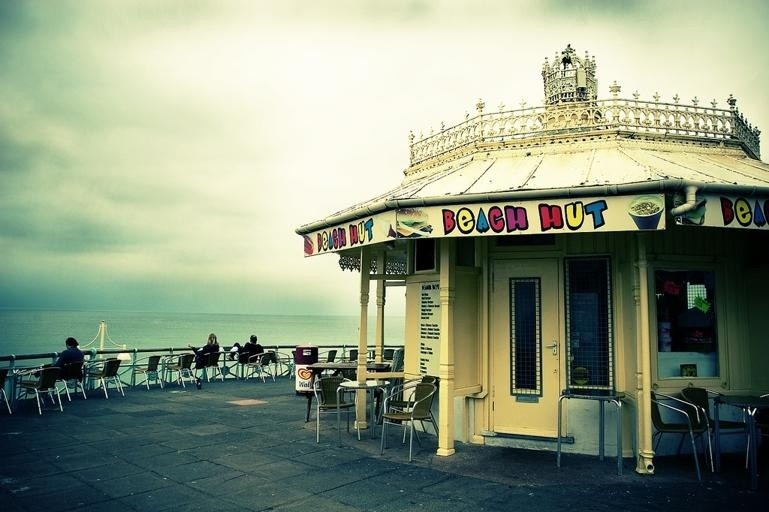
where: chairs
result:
[0,350,291,415]
[651,386,769,480]
[307,349,439,462]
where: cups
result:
[627,194,664,230]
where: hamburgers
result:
[378,208,439,239]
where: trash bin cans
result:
[294,346,318,396]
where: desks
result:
[557,388,626,475]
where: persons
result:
[227,335,264,364]
[188,333,221,369]
[33,337,84,404]
